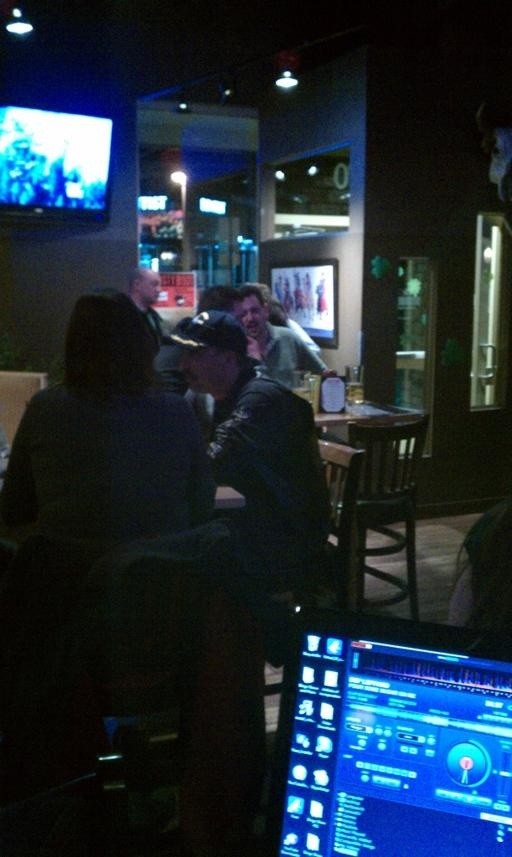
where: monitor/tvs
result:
[272,602,512,851]
[1,98,119,227]
[268,258,338,349]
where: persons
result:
[159,310,333,580]
[445,500,511,631]
[0,292,218,537]
[154,282,329,424]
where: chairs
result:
[330,414,430,621]
[259,438,368,752]
[0,370,47,445]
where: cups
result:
[289,368,321,416]
[343,364,367,405]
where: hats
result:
[169,309,250,349]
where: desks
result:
[215,484,245,512]
[312,410,424,428]
